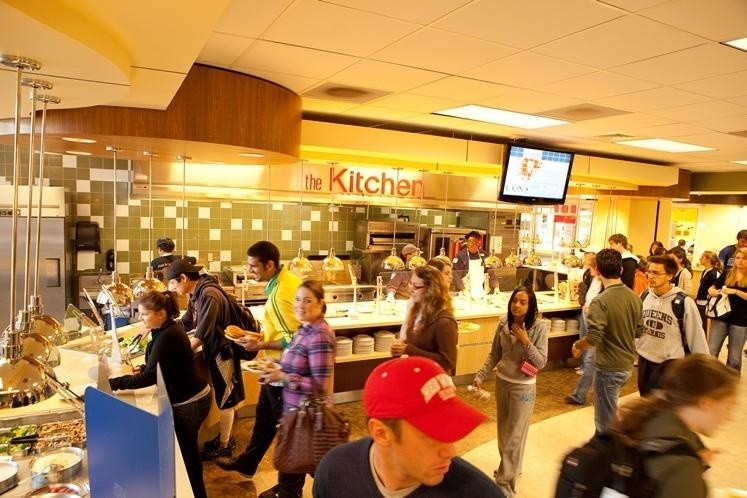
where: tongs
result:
[11,428,65,444]
[122,334,142,353]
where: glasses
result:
[646,269,667,277]
[407,282,426,290]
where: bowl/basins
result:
[0,460,17,494]
[19,483,89,498]
[28,447,84,482]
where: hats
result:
[363,356,485,443]
[167,259,203,280]
[580,245,601,254]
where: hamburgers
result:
[225,324,245,338]
[245,329,263,343]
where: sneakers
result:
[200,432,236,463]
[216,456,255,478]
[258,485,302,498]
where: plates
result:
[457,323,480,334]
[240,360,283,374]
[543,317,580,333]
[336,330,400,358]
[224,330,264,343]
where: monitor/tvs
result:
[498,141,575,206]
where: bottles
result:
[466,385,491,400]
[572,344,580,359]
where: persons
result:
[391,265,458,378]
[259,281,336,497]
[215,241,302,480]
[614,356,739,497]
[638,256,708,400]
[452,231,499,295]
[385,244,451,302]
[105,238,258,498]
[312,357,507,498]
[472,286,548,498]
[572,250,644,433]
[607,231,692,299]
[696,228,747,370]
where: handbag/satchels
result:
[274,398,350,474]
[519,360,539,377]
[704,286,732,318]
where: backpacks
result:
[198,282,260,361]
[556,429,700,497]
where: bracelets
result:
[735,289,738,295]
